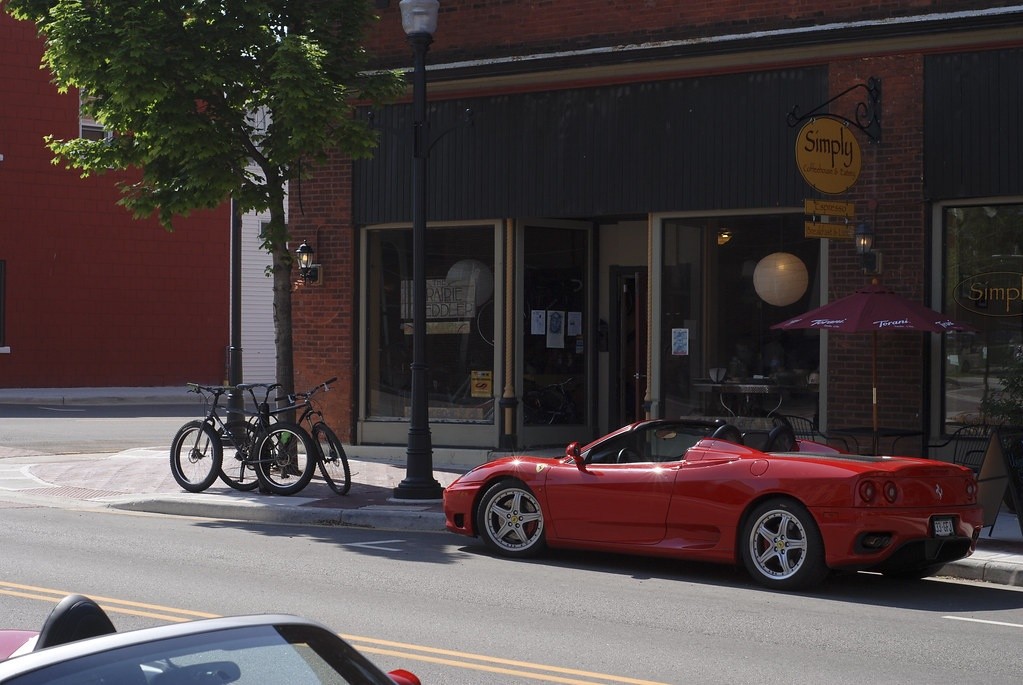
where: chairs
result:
[772,415,859,453]
[766,426,799,453]
[713,424,743,446]
[922,424,994,471]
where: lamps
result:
[717,224,732,249]
[853,217,882,276]
[295,240,318,286]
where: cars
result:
[0,594,422,685]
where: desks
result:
[831,427,924,455]
[692,382,808,418]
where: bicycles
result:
[170,376,351,496]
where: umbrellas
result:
[769,285,961,455]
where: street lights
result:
[394,0,445,500]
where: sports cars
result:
[442,420,983,591]
